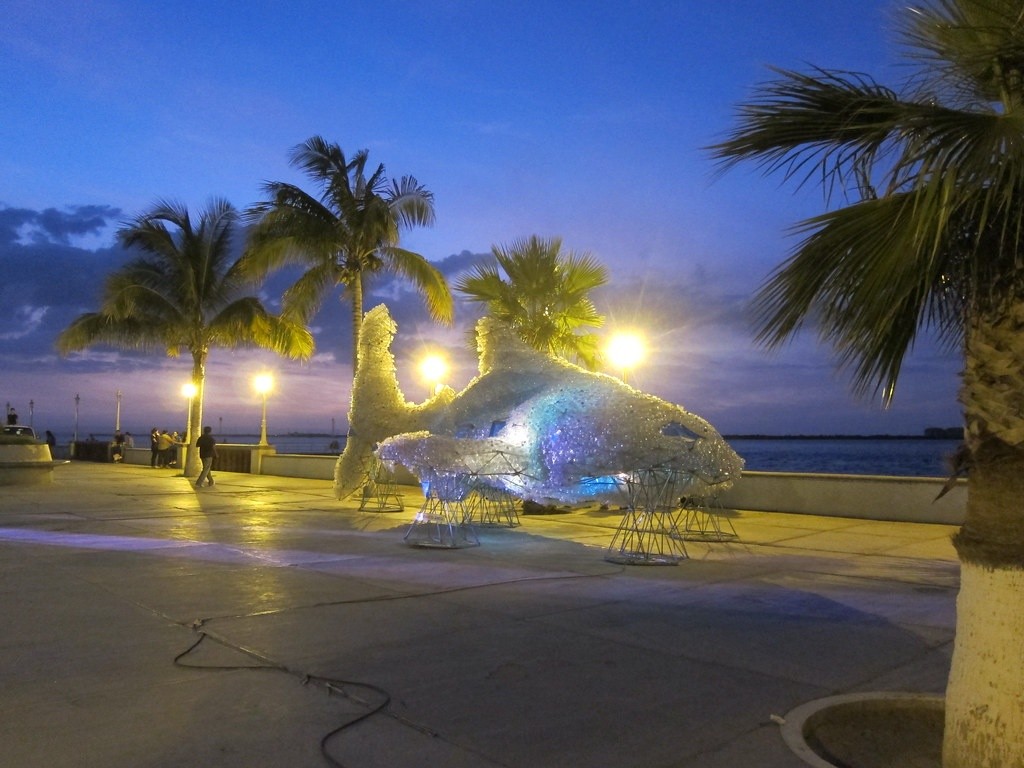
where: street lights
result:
[251,372,275,446]
[115,390,122,433]
[180,381,196,443]
[69,393,80,441]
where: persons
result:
[108,429,135,464]
[194,426,216,488]
[89,433,98,441]
[45,430,56,460]
[150,427,187,469]
[7,408,18,426]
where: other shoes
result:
[159,464,163,468]
[620,505,629,510]
[195,483,204,488]
[209,480,215,488]
[152,465,160,469]
[601,505,609,510]
[166,465,171,469]
